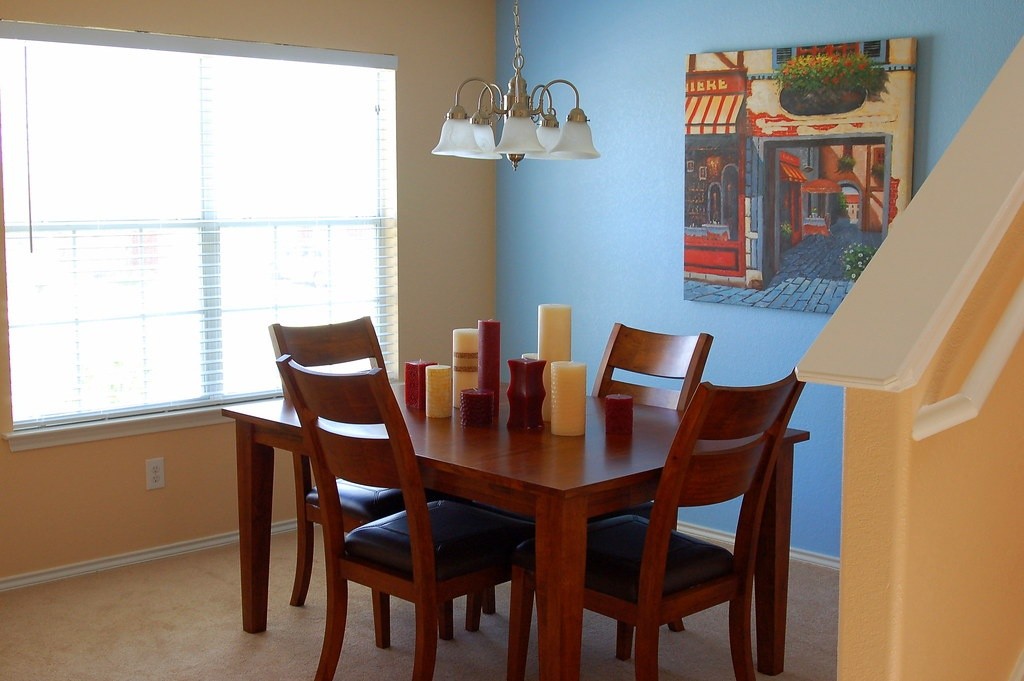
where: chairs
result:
[507,368,807,681]
[268,316,495,649]
[464,324,714,635]
[276,353,536,681]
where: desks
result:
[221,382,810,681]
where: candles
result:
[506,358,547,429]
[452,328,478,409]
[550,360,587,436]
[459,386,494,427]
[425,363,453,418]
[604,392,634,435]
[477,318,500,416]
[538,303,572,421]
[404,358,438,410]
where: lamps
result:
[430,0,601,172]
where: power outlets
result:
[144,456,166,491]
